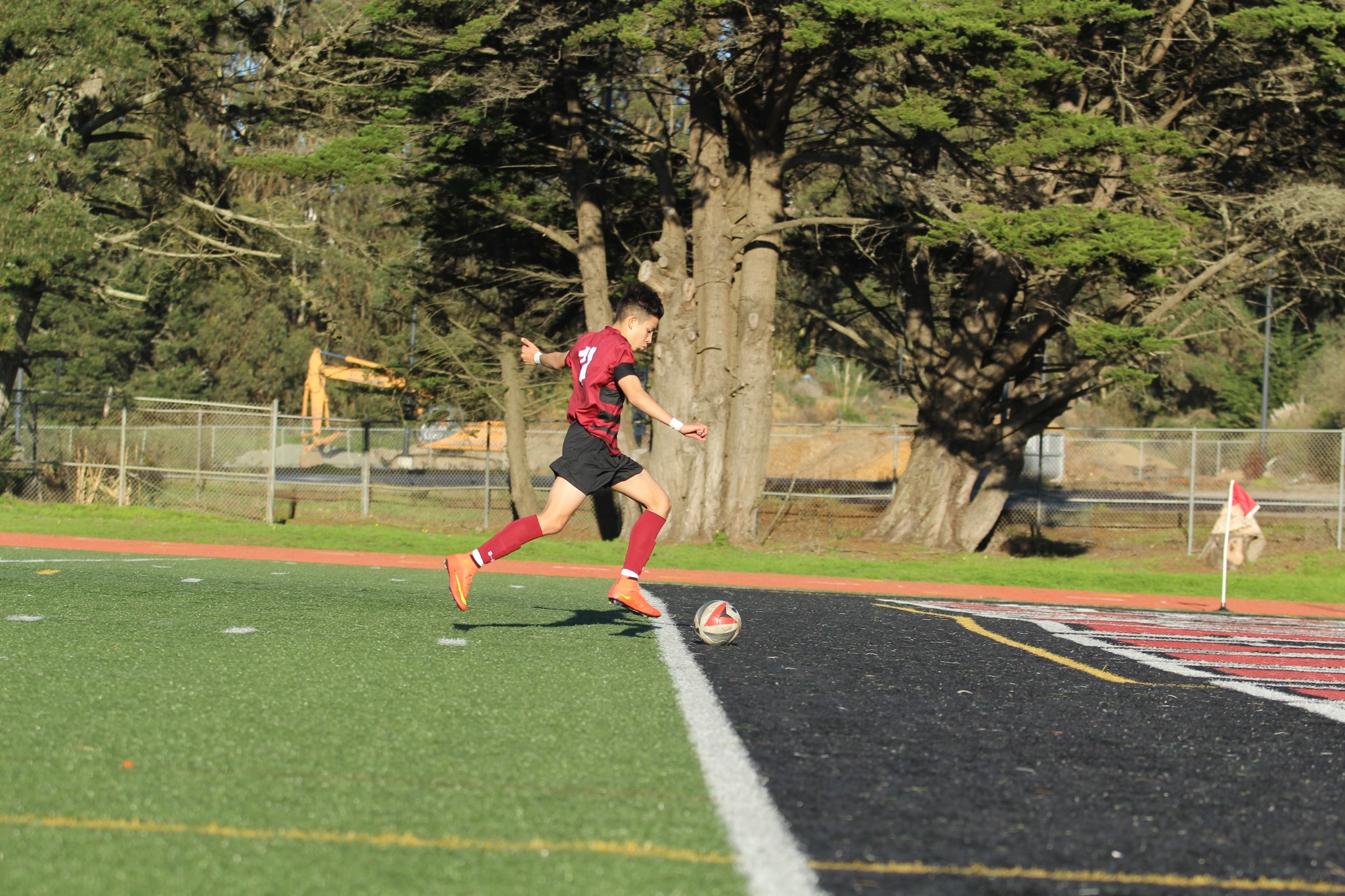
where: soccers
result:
[693,599,742,646]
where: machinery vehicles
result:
[297,349,506,468]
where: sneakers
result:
[608,575,661,618]
[443,550,481,612]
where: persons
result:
[442,280,710,619]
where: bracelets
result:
[534,351,542,365]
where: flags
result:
[1228,480,1261,520]
